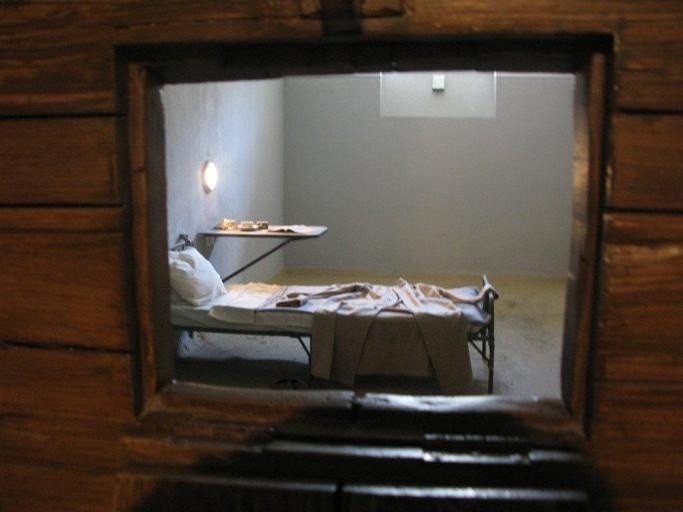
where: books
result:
[269,224,311,235]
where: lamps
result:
[203,160,218,192]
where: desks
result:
[198,224,329,283]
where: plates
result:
[239,224,257,231]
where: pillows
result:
[168,246,227,306]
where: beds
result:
[169,234,499,394]
